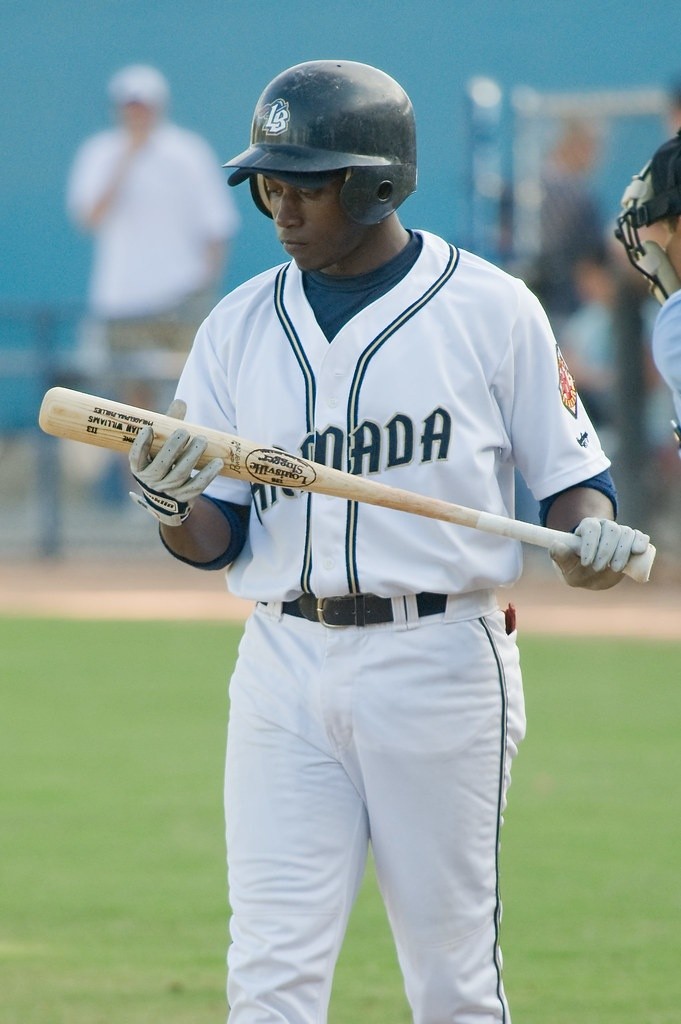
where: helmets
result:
[222,59,418,226]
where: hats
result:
[110,66,166,107]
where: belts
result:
[256,592,447,628]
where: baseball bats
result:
[34,386,658,585]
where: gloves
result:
[548,517,650,591]
[129,399,223,528]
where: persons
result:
[69,66,241,507]
[497,108,680,531]
[129,60,651,1024]
